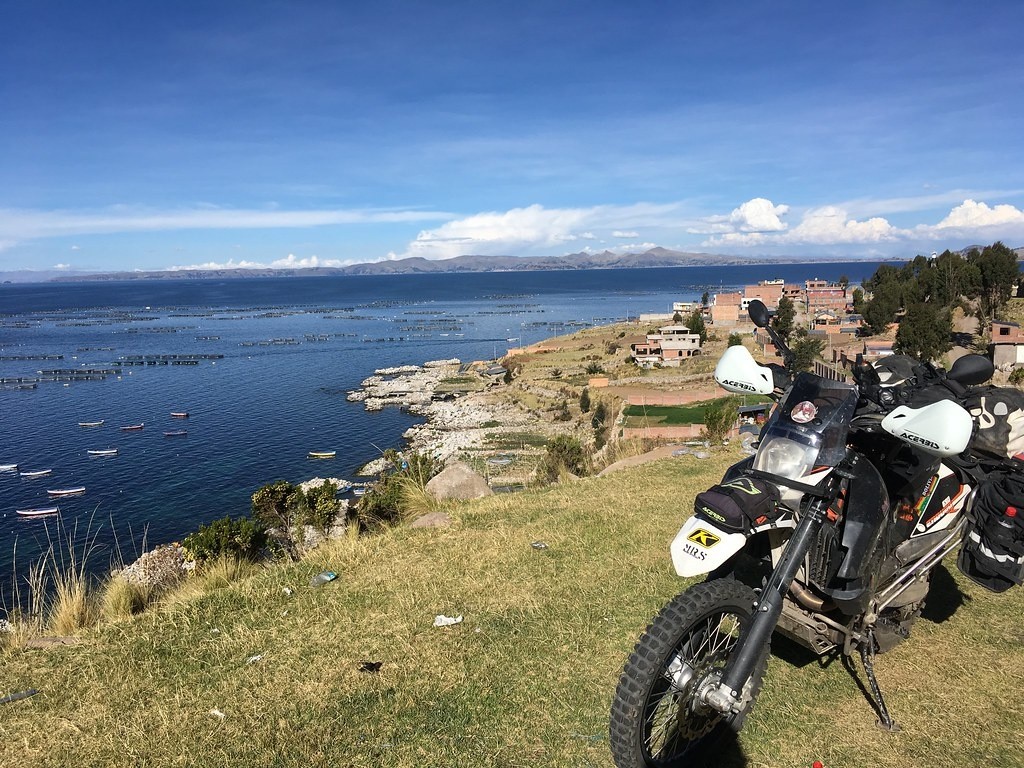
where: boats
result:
[21,468,51,476]
[79,419,104,426]
[352,487,371,495]
[87,449,118,454]
[310,450,337,456]
[169,412,188,417]
[120,424,142,429]
[48,487,85,496]
[164,431,188,436]
[1,465,16,468]
[16,507,59,515]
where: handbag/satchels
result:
[871,352,919,389]
[963,385,1024,460]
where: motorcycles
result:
[607,296,1024,768]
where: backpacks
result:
[956,470,1024,593]
